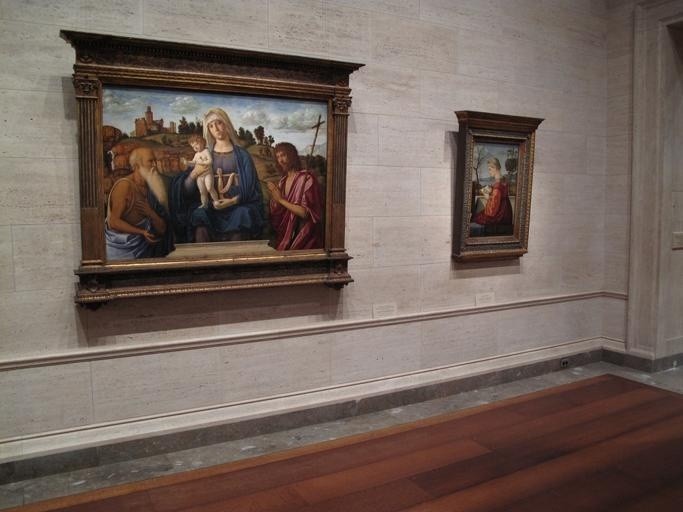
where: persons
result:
[470,158,512,225]
[105,107,321,259]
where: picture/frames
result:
[53,27,366,312]
[446,105,547,264]
